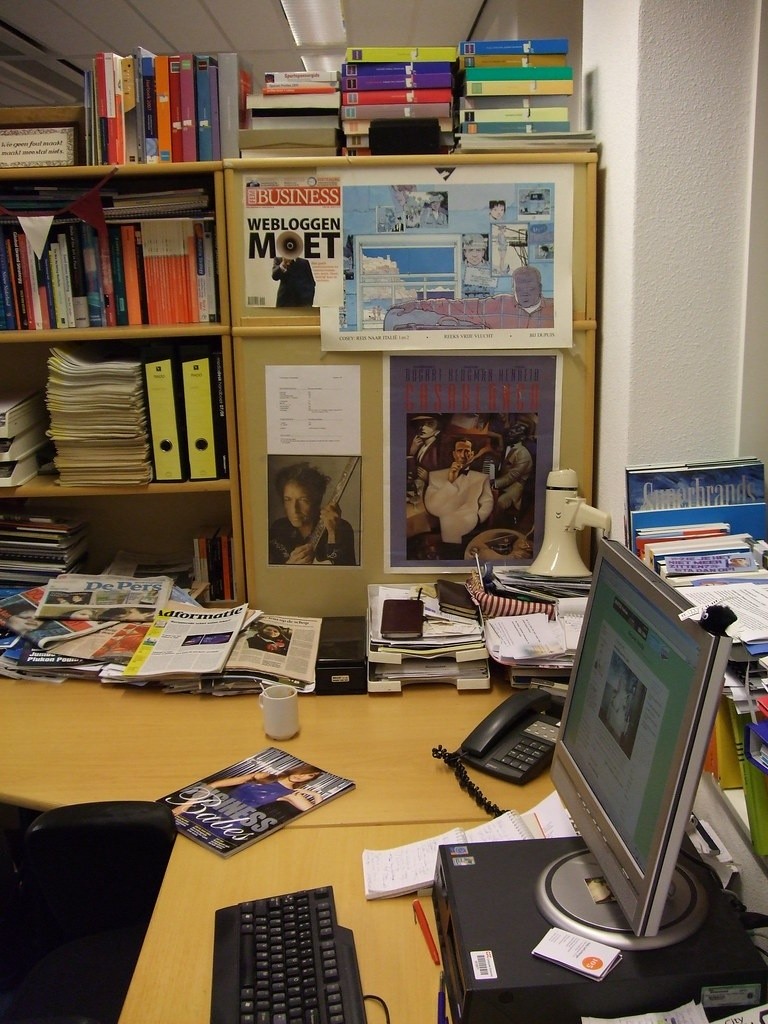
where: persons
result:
[273,257,314,308]
[268,465,354,562]
[0,603,43,631]
[57,595,67,605]
[70,595,86,605]
[495,415,535,526]
[248,625,286,654]
[174,764,324,849]
[424,437,492,557]
[409,416,449,488]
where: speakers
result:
[368,117,441,156]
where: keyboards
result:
[209,885,367,1024]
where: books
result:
[0,33,768,901]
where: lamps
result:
[529,468,612,578]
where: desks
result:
[116,821,488,1024]
[0,673,557,828]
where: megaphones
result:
[276,231,303,259]
[526,469,611,577]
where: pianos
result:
[477,448,502,495]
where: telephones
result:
[454,688,567,787]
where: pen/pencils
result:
[410,898,441,966]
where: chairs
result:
[0,801,176,1024]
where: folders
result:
[141,341,227,483]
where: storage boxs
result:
[0,391,55,488]
[367,117,442,155]
[315,617,368,696]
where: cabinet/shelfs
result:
[0,161,246,608]
[219,153,602,616]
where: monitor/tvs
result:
[535,538,734,951]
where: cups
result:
[257,685,300,739]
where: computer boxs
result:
[432,836,768,1024]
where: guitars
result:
[283,454,362,568]
